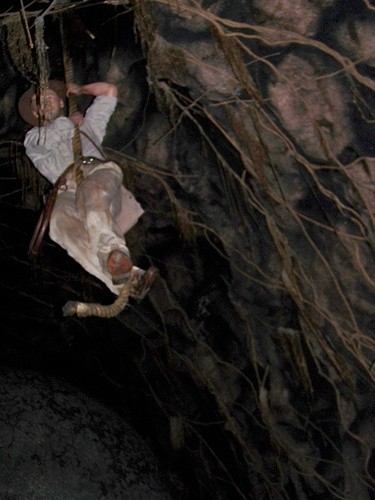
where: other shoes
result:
[132,266,160,303]
[106,248,133,286]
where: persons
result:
[18,79,158,301]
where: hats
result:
[18,80,67,126]
